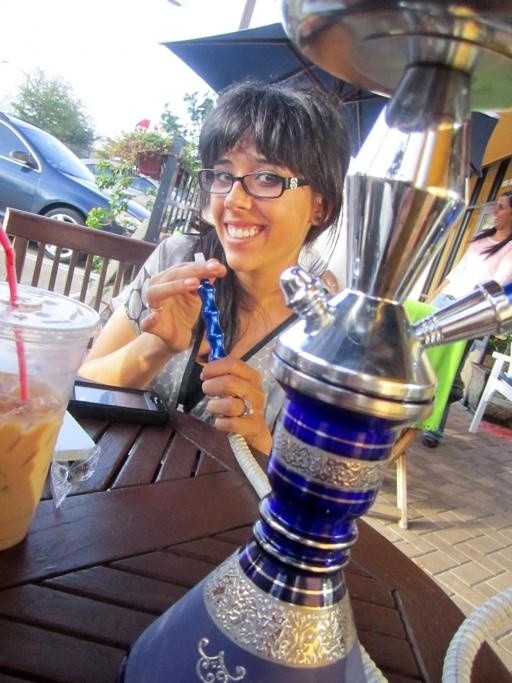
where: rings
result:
[238,394,256,420]
[145,302,166,312]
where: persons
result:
[420,180,512,312]
[74,78,355,459]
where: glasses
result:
[196,168,309,200]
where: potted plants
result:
[73,125,198,270]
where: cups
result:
[0,281,103,551]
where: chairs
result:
[385,302,466,528]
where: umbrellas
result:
[158,16,502,203]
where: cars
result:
[0,112,185,263]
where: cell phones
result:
[67,380,169,427]
